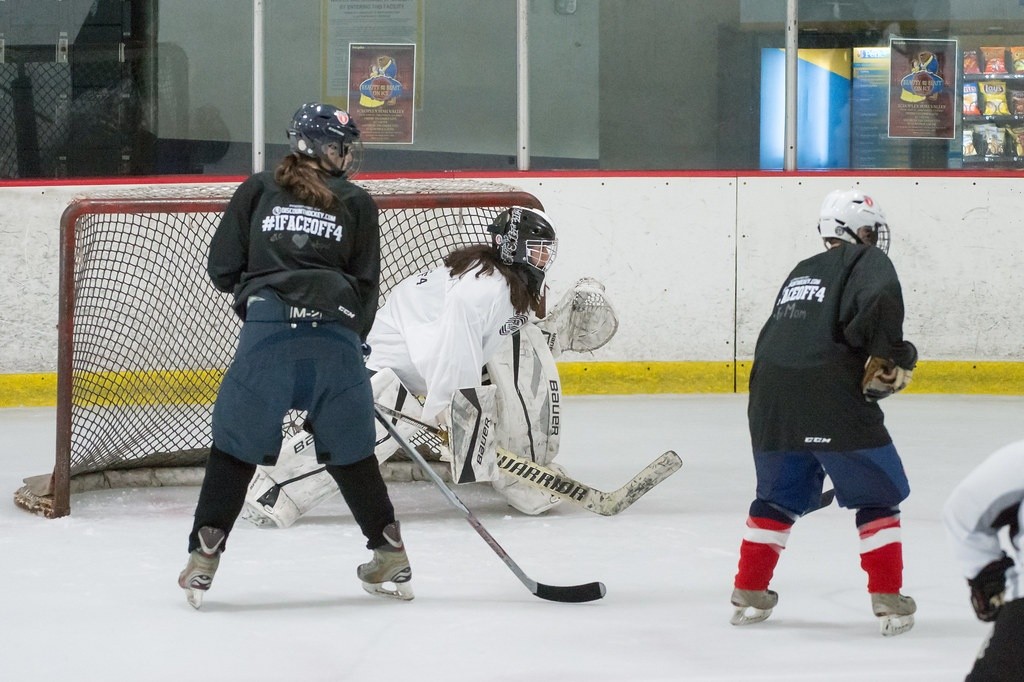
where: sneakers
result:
[356,520,416,601]
[178,526,227,611]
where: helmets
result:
[819,190,890,256]
[288,102,362,173]
[488,208,556,273]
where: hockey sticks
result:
[374,403,682,517]
[374,405,606,603]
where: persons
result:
[723,186,924,620]
[242,202,575,524]
[952,451,1024,681]
[172,102,414,583]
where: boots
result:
[730,497,804,627]
[242,368,428,528]
[486,322,560,516]
[854,501,917,637]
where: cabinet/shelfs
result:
[948,34,1024,170]
